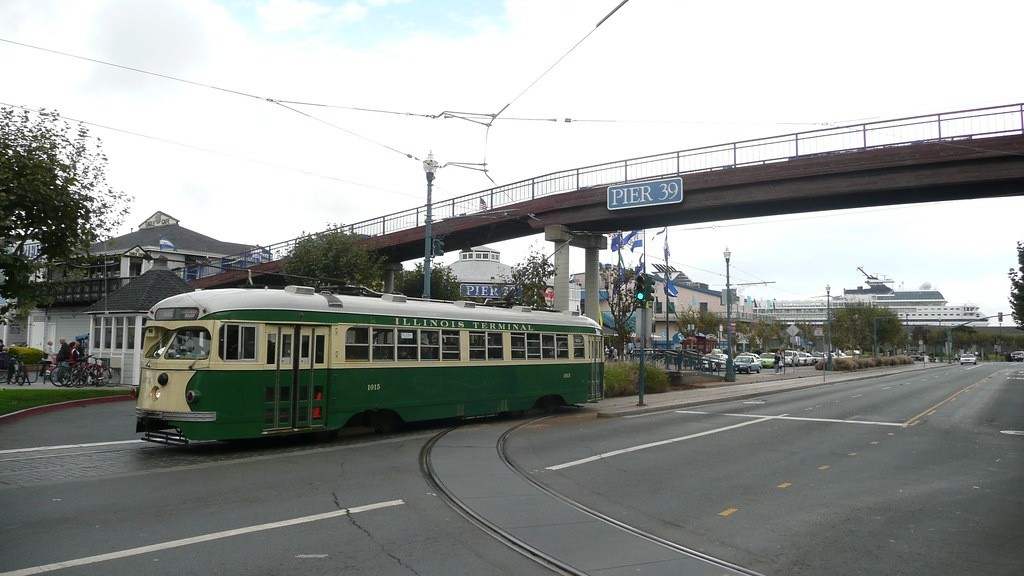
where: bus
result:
[136,284,606,447]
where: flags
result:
[159,234,177,252]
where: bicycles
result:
[49,354,121,388]
[6,355,26,386]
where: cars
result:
[826,350,860,360]
[960,352,977,365]
[694,353,729,371]
[812,353,828,363]
[738,352,763,364]
[760,353,776,369]
[733,356,762,374]
[798,353,814,366]
[785,350,800,367]
[1011,352,1023,359]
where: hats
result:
[422,338,429,345]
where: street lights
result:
[938,317,943,362]
[826,284,833,371]
[421,150,439,301]
[723,246,736,382]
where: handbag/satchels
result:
[779,361,783,367]
[43,353,48,359]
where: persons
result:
[604,346,618,363]
[384,333,460,360]
[0,339,16,379]
[903,350,916,356]
[39,337,85,384]
[953,353,959,363]
[984,351,989,362]
[774,350,784,375]
[470,338,550,359]
[629,346,663,361]
[791,353,799,368]
[267,340,274,364]
[251,245,262,264]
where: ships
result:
[752,267,991,327]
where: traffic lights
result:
[645,278,655,302]
[636,276,645,300]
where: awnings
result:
[601,311,637,331]
[75,333,90,340]
[581,289,609,299]
